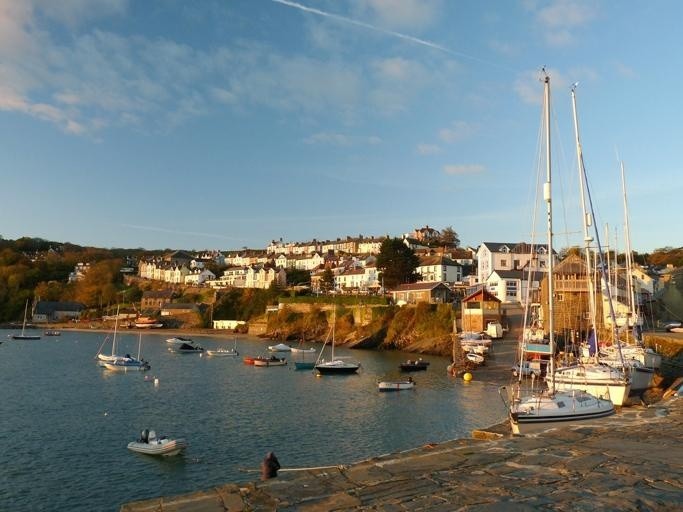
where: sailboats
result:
[13,299,40,340]
[311,303,361,374]
[98,304,142,363]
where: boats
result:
[165,335,194,345]
[207,334,239,356]
[377,376,416,391]
[43,329,61,336]
[268,330,316,369]
[168,343,204,353]
[102,361,150,372]
[127,437,188,458]
[243,354,287,366]
[399,358,429,370]
[458,327,493,366]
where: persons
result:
[261,451,280,481]
